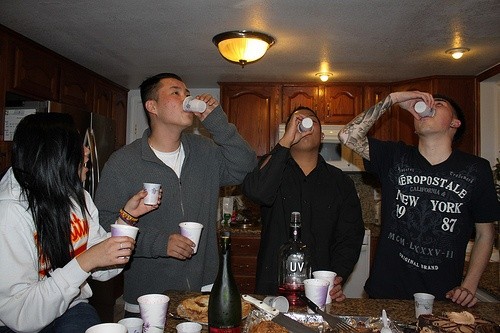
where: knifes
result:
[241,294,322,333]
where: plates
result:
[176,292,252,325]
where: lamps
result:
[315,72,333,83]
[212,29,276,65]
[445,48,470,59]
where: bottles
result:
[280,212,311,308]
[207,229,243,333]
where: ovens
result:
[279,124,366,173]
[340,229,372,298]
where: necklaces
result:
[147,136,180,170]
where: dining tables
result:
[131,293,500,333]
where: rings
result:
[120,242,123,249]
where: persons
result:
[244,104,365,304]
[0,112,162,333]
[339,92,499,307]
[94,73,259,319]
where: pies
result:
[182,293,251,324]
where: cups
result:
[413,101,435,118]
[303,278,330,314]
[263,294,290,314]
[176,322,203,333]
[110,223,140,261]
[182,95,207,113]
[84,322,128,333]
[178,221,204,253]
[117,317,143,333]
[137,294,170,333]
[143,182,161,206]
[312,270,338,303]
[298,116,314,135]
[413,292,435,319]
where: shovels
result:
[379,308,393,333]
[301,295,359,333]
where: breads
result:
[255,320,290,333]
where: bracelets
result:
[119,209,138,227]
